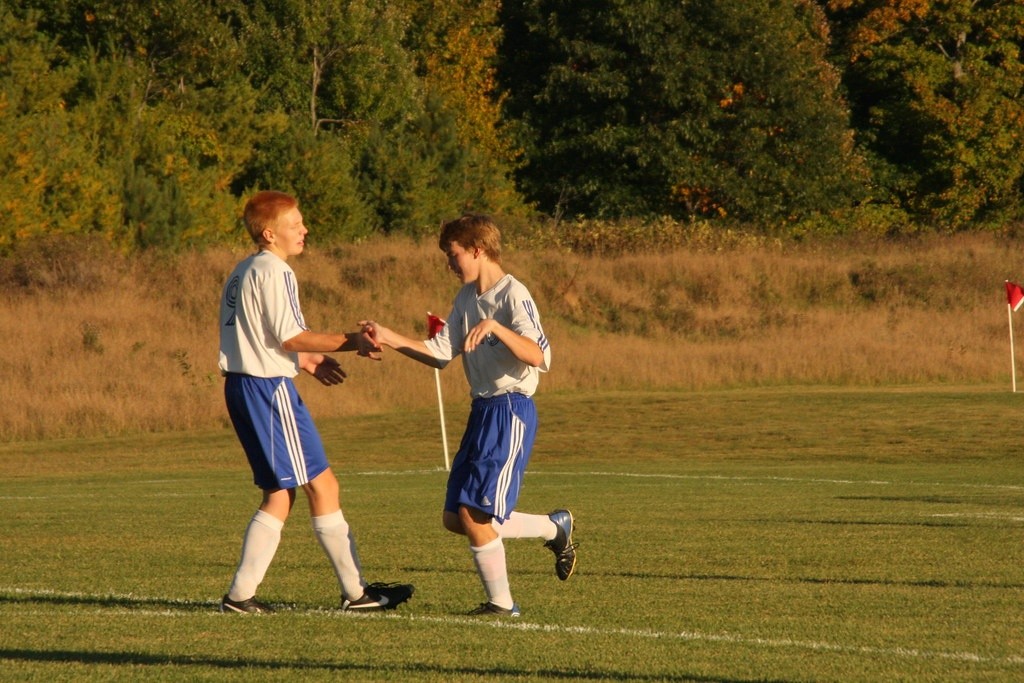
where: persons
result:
[356,216,576,618]
[216,191,414,615]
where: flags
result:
[1006,281,1024,312]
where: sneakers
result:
[465,600,521,617]
[220,594,278,617]
[543,509,580,582]
[340,580,415,612]
